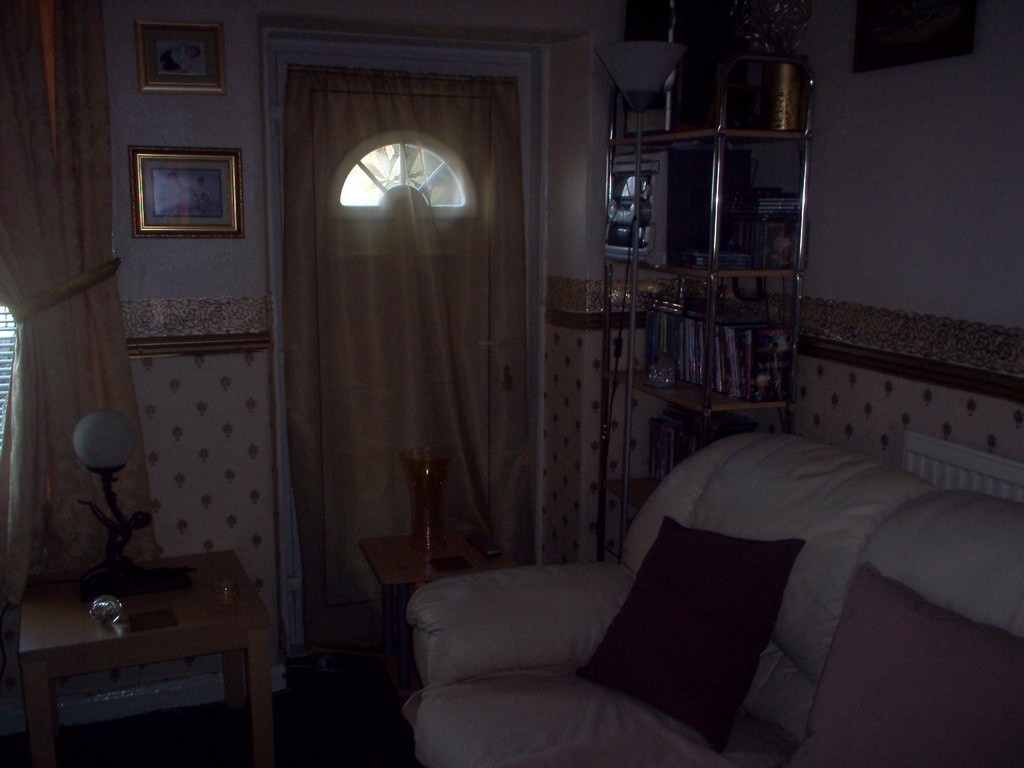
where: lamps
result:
[600,41,682,560]
[72,409,153,603]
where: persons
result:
[159,172,215,217]
[157,43,201,72]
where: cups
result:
[397,448,453,551]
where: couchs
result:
[401,434,1024,768]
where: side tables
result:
[361,530,520,700]
[19,550,270,768]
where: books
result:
[645,312,793,403]
[648,405,758,482]
[694,193,801,272]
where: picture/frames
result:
[128,144,244,239]
[133,17,227,95]
[852,0,979,74]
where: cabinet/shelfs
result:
[597,53,815,563]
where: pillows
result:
[575,515,806,754]
[790,562,1024,768]
